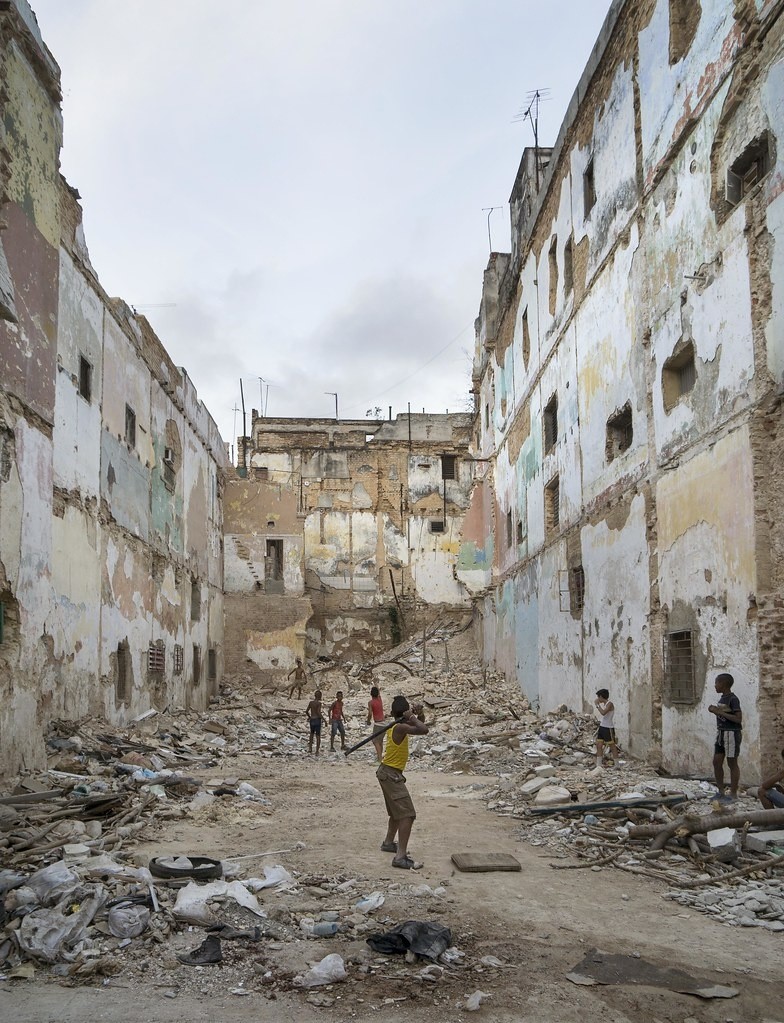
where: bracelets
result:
[419,713,423,716]
[409,715,413,718]
[596,705,600,708]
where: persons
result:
[288,661,307,700]
[758,750,784,810]
[595,689,620,771]
[377,696,429,869]
[306,691,327,752]
[707,673,742,804]
[328,691,350,752]
[367,676,386,766]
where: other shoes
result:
[341,745,350,749]
[711,792,726,799]
[315,750,319,754]
[720,795,738,803]
[307,749,312,753]
[330,747,336,752]
[392,854,423,870]
[381,841,399,852]
